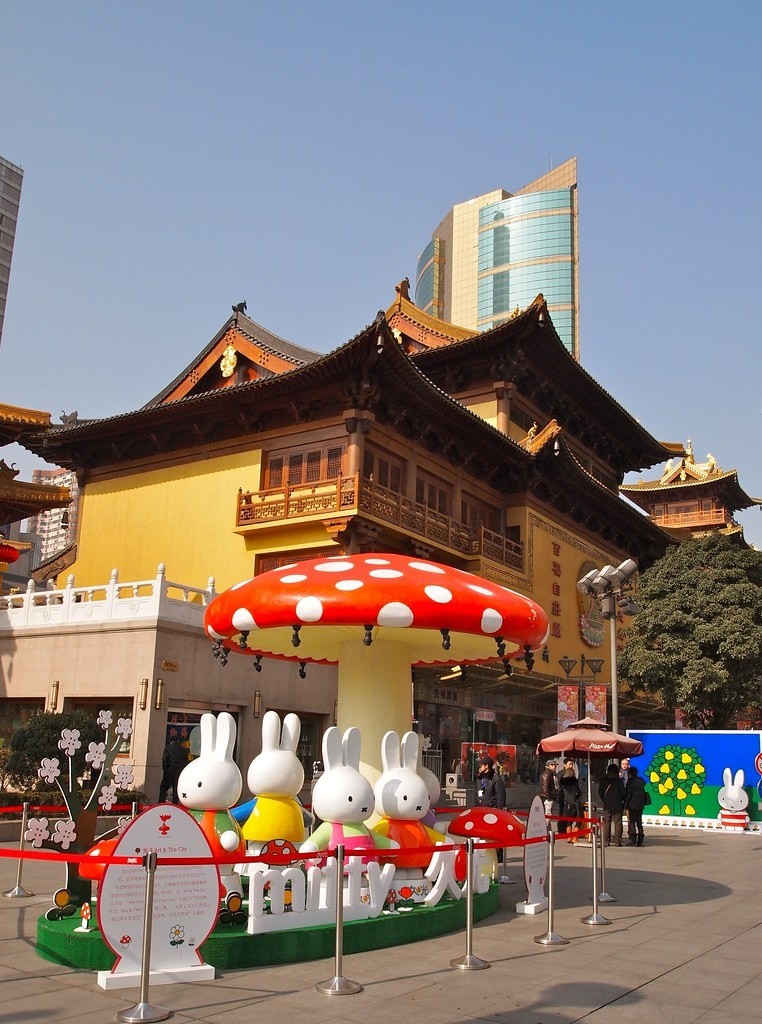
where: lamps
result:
[254,690,261,717]
[156,679,165,709]
[51,680,59,709]
[334,700,338,724]
[140,678,148,709]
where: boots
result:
[566,826,572,843]
[636,833,645,847]
[626,834,638,846]
[571,827,579,844]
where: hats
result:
[479,757,493,764]
[546,760,558,765]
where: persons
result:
[476,757,506,863]
[539,758,582,843]
[597,758,645,847]
[158,736,188,804]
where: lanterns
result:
[0,544,19,574]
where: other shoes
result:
[615,843,623,847]
[604,844,609,847]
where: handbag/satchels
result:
[644,792,651,805]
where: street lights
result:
[576,559,641,771]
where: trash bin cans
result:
[578,802,597,839]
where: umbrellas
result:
[536,717,644,842]
